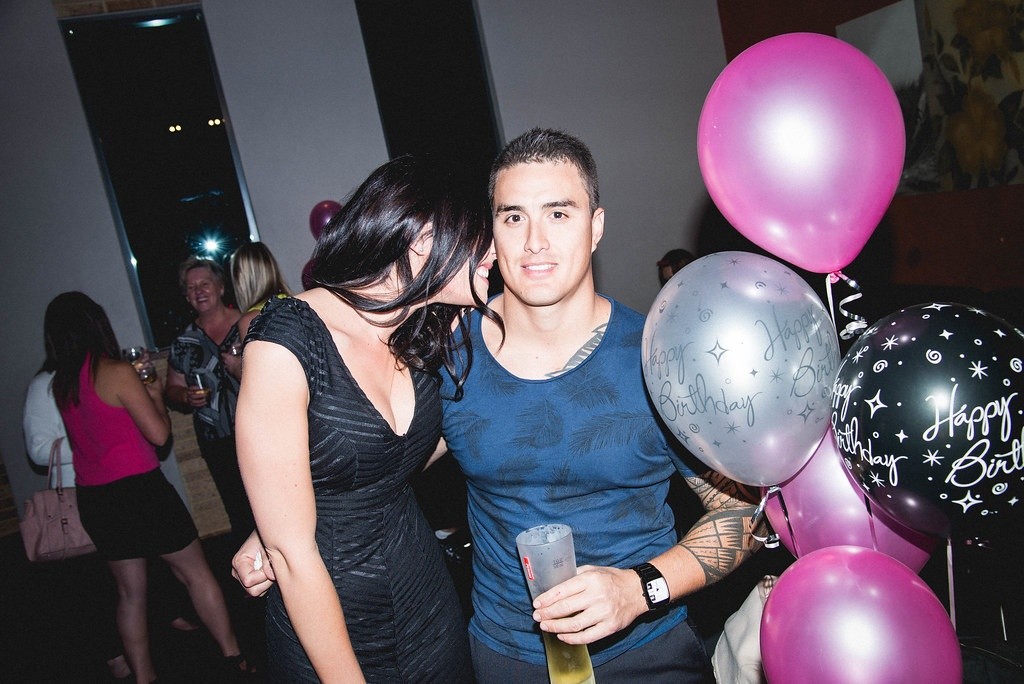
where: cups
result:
[123,346,158,387]
[517,522,598,684]
[224,344,240,363]
[184,371,212,409]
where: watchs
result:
[624,563,671,612]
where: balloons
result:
[761,428,932,574]
[696,31,905,274]
[642,248,839,496]
[309,200,342,242]
[830,302,1024,547]
[760,545,963,684]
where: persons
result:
[22,240,292,684]
[655,249,695,284]
[233,149,497,684]
[230,128,772,684]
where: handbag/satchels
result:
[21,436,99,560]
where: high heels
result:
[221,654,266,680]
